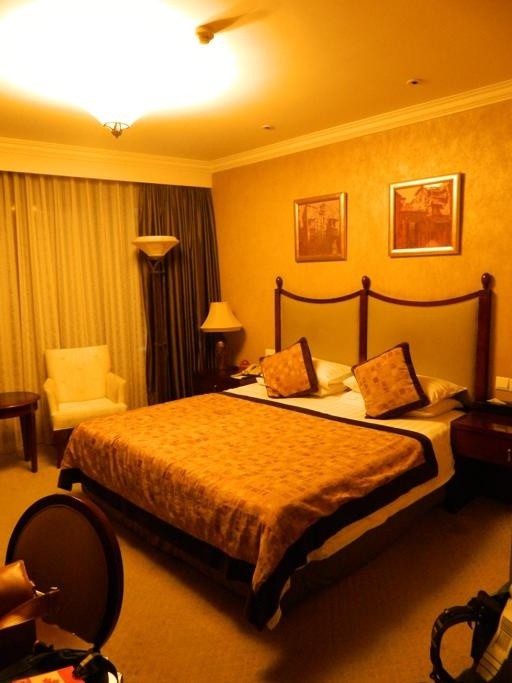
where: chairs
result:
[427,579,512,683]
[3,492,123,649]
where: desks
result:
[0,391,41,473]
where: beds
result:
[60,272,493,636]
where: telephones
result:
[231,362,262,377]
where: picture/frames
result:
[388,173,461,257]
[293,191,347,262]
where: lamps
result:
[131,235,179,273]
[200,301,243,370]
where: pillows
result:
[339,391,461,419]
[341,375,465,406]
[255,377,348,398]
[312,356,354,388]
[259,336,319,399]
[351,341,430,419]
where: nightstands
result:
[448,410,512,504]
[190,366,257,397]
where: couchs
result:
[42,344,128,469]
